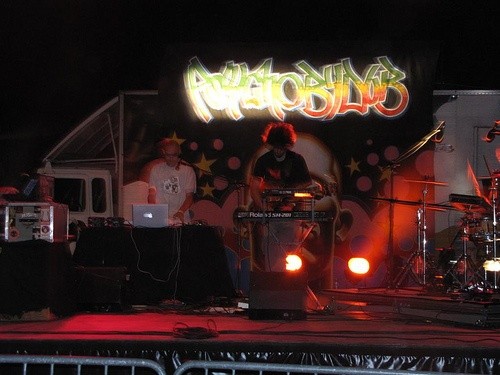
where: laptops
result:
[132,204,169,228]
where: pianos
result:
[234,188,335,312]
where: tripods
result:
[359,131,500,293]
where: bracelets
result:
[177,210,184,213]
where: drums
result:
[463,215,500,287]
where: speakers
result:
[65,267,128,311]
[249,272,308,319]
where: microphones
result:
[435,122,446,142]
[175,157,183,171]
[486,120,500,142]
[450,229,462,246]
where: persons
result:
[250,125,311,269]
[147,140,197,225]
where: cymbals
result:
[403,179,448,185]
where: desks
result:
[71,225,236,307]
[0,238,80,321]
